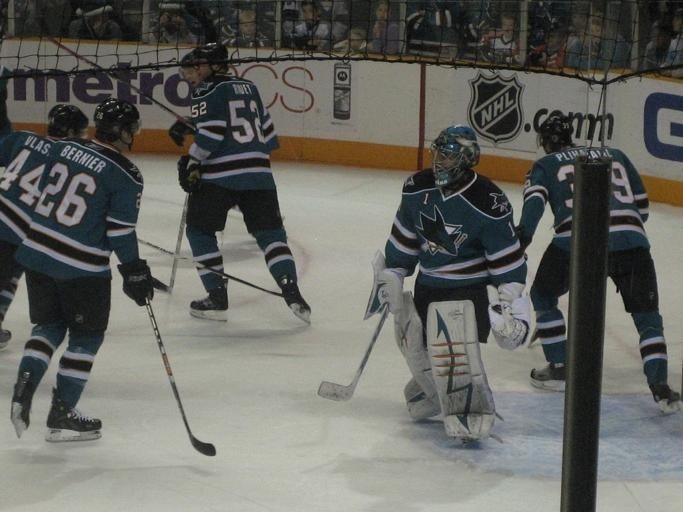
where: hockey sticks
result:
[317,302,388,401]
[145,297,216,458]
[35,10,197,131]
[152,191,191,293]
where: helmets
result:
[48,104,88,131]
[428,126,481,186]
[181,42,228,68]
[93,98,141,136]
[538,115,574,153]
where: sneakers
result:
[649,384,681,406]
[1,329,11,348]
[12,370,37,428]
[277,274,310,312]
[46,388,101,433]
[190,278,228,310]
[531,363,566,381]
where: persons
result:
[169,41,312,324]
[11,97,167,441]
[378,127,531,446]
[1,103,90,347]
[514,109,683,415]
[1,2,683,80]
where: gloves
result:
[168,120,188,147]
[117,259,154,306]
[178,155,200,193]
